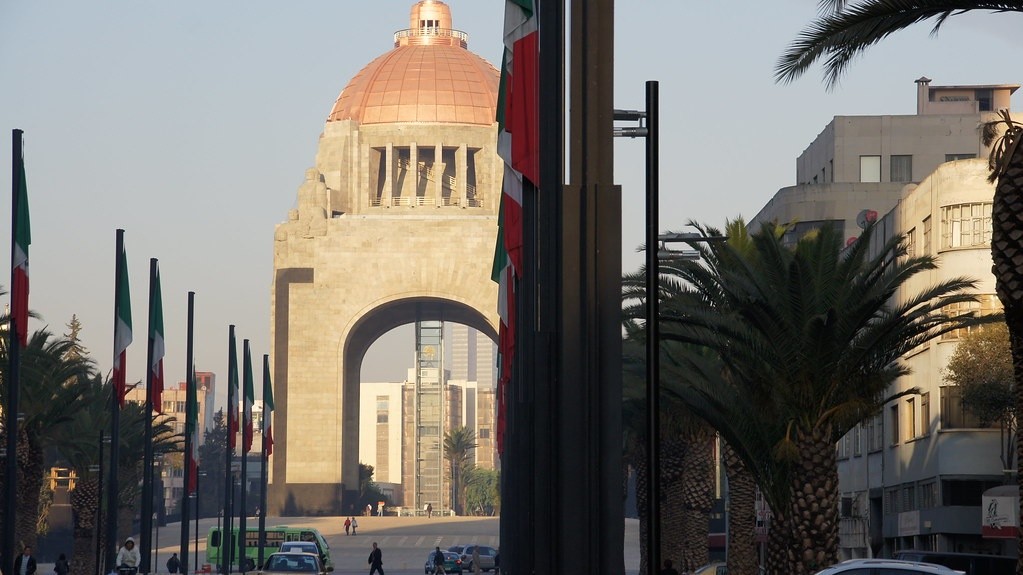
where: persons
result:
[366,505,371,517]
[256,508,260,517]
[166,553,182,575]
[117,537,140,575]
[472,545,481,575]
[377,502,385,517]
[344,518,357,536]
[368,542,385,575]
[55,553,68,575]
[433,547,447,575]
[495,546,500,575]
[14,546,37,575]
[424,503,432,518]
[249,541,279,547]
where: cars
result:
[425,550,464,575]
[257,551,334,575]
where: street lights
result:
[610,106,668,575]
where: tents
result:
[113,237,132,412]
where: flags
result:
[149,259,165,415]
[11,142,31,349]
[187,365,199,494]
[227,337,275,457]
[497,0,539,454]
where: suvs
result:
[448,544,499,572]
[815,556,967,575]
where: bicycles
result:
[117,566,139,575]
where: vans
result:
[280,541,320,554]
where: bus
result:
[894,550,1020,575]
[206,525,331,571]
